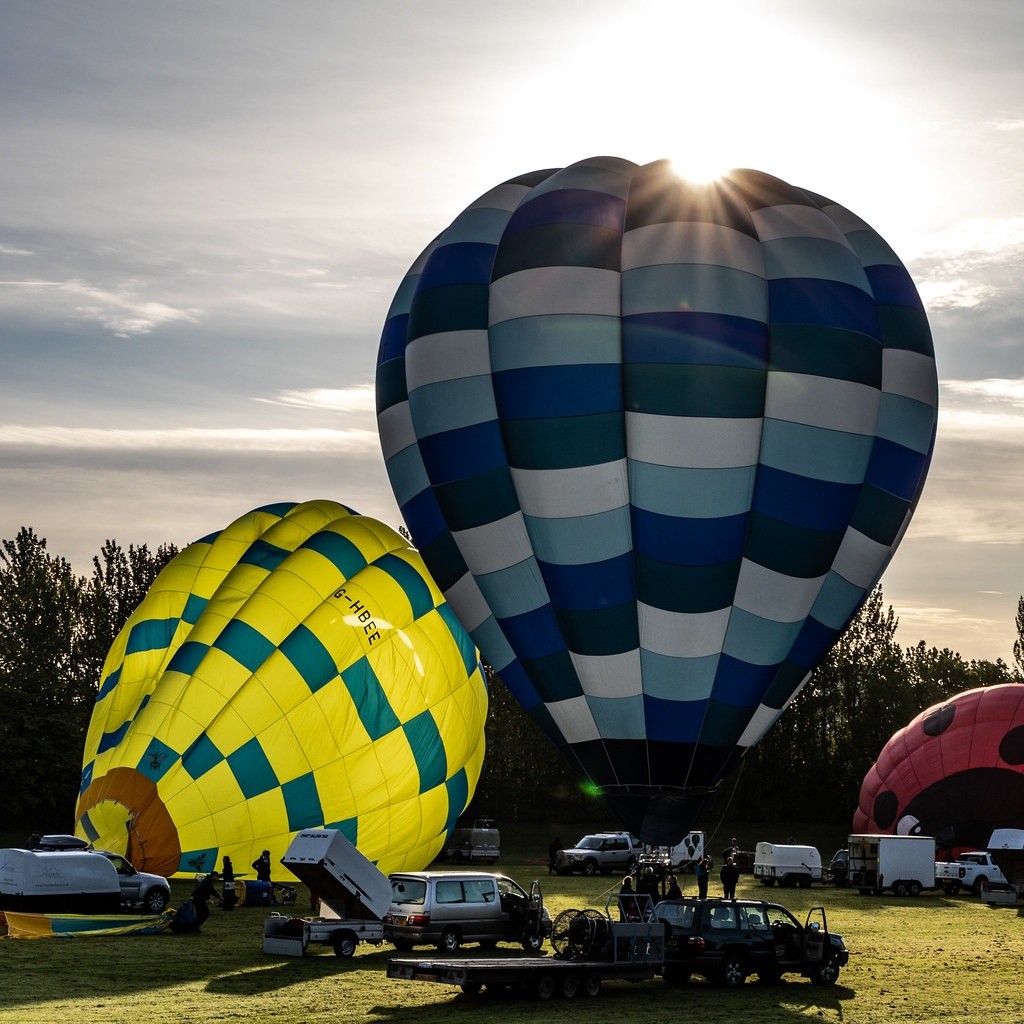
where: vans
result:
[382,871,552,951]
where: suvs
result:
[557,832,637,877]
[31,835,173,916]
[646,894,849,990]
[945,853,1009,900]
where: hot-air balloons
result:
[73,499,489,883]
[856,681,1024,855]
[377,158,941,916]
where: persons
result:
[222,855,237,912]
[252,849,280,906]
[544,832,745,925]
[190,870,227,935]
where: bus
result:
[446,829,501,865]
